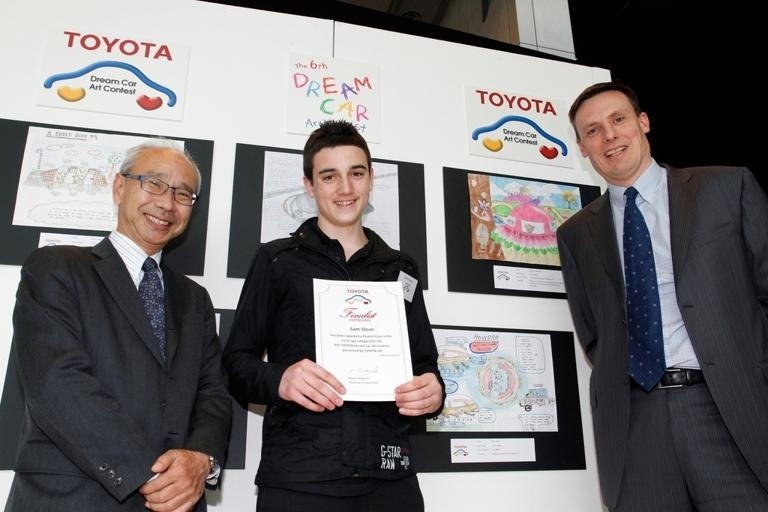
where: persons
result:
[3,138,234,512]
[222,120,447,512]
[556,82,767,512]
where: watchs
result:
[209,456,215,474]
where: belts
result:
[629,367,705,390]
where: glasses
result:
[123,174,200,209]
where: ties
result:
[138,257,166,365]
[623,187,667,393]
